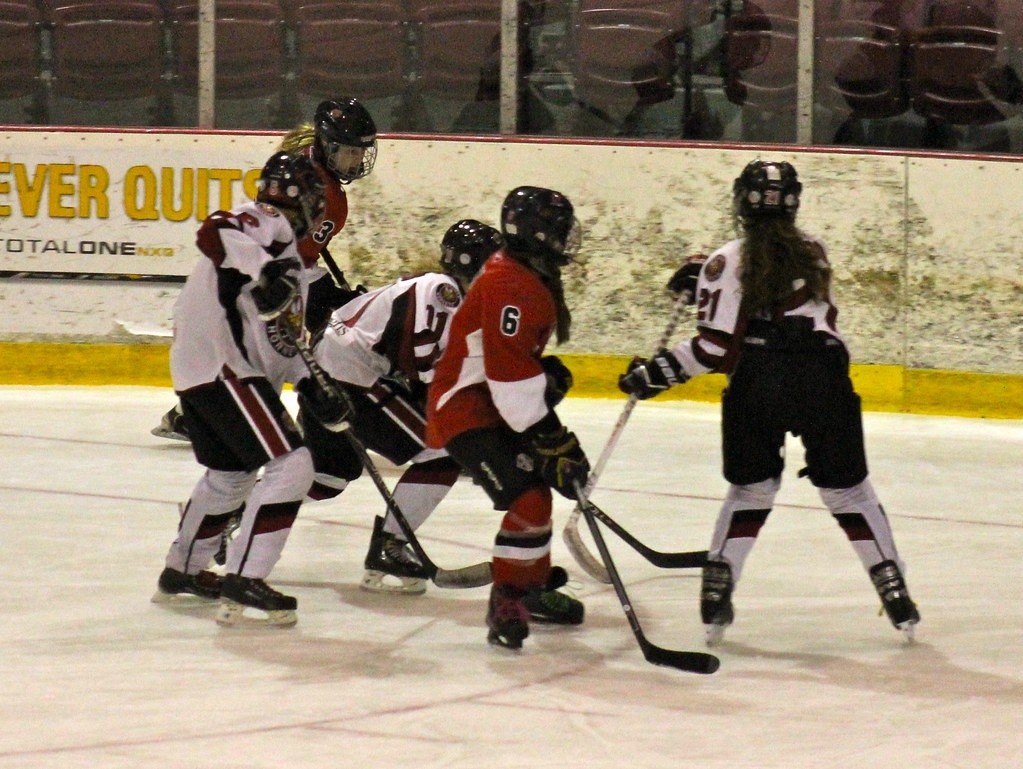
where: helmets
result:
[500,187,582,266]
[315,98,379,181]
[440,218,505,280]
[731,159,801,223]
[257,152,325,236]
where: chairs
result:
[0,0,1023,149]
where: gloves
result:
[299,370,352,434]
[668,261,703,304]
[252,276,306,335]
[520,415,592,500]
[617,351,687,400]
[306,271,363,331]
[537,355,575,410]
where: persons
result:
[831,0,1022,160]
[429,185,591,651]
[177,219,500,595]
[151,150,328,626]
[450,0,581,137]
[619,0,773,143]
[152,98,378,440]
[618,159,921,647]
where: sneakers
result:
[215,572,298,625]
[487,560,529,649]
[699,559,735,649]
[359,516,427,595]
[149,563,224,608]
[868,560,920,644]
[520,567,584,625]
[151,407,193,441]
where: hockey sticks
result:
[587,498,710,571]
[561,289,693,584]
[574,477,722,675]
[280,308,494,589]
[317,235,350,292]
